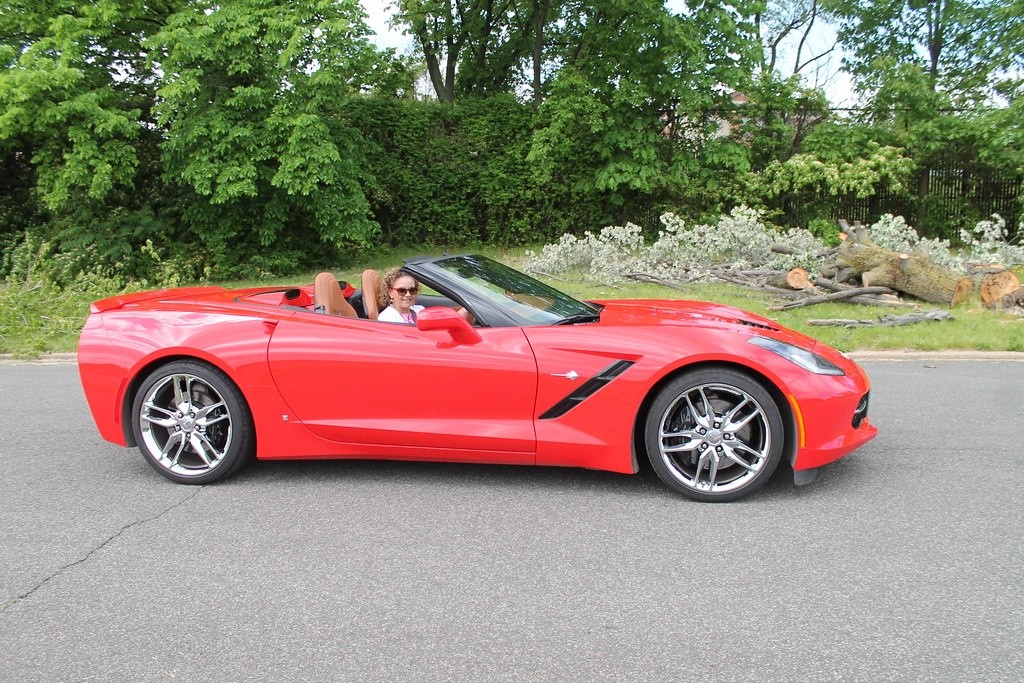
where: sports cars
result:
[75,251,883,505]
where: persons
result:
[377,268,427,323]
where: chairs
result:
[314,272,358,316]
[361,269,382,320]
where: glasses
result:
[389,287,417,296]
